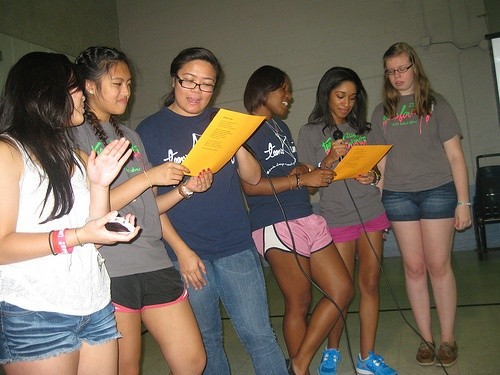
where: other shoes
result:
[285,357,295,375]
[436,341,458,366]
[416,340,436,365]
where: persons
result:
[370,42,472,368]
[240,65,354,375]
[298,67,400,375]
[134,47,290,375]
[63,46,214,375]
[0,51,141,375]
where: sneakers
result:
[355,351,398,375]
[317,344,340,375]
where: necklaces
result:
[265,119,283,134]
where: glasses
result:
[175,75,215,92]
[385,64,413,75]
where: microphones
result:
[211,111,250,150]
[332,130,344,161]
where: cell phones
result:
[104,222,130,233]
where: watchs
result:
[179,182,193,199]
[371,168,381,187]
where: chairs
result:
[472,153,500,260]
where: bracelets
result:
[296,173,302,190]
[144,170,153,188]
[322,161,334,170]
[53,228,73,256]
[73,228,83,247]
[456,202,471,206]
[288,174,294,190]
[48,230,58,256]
